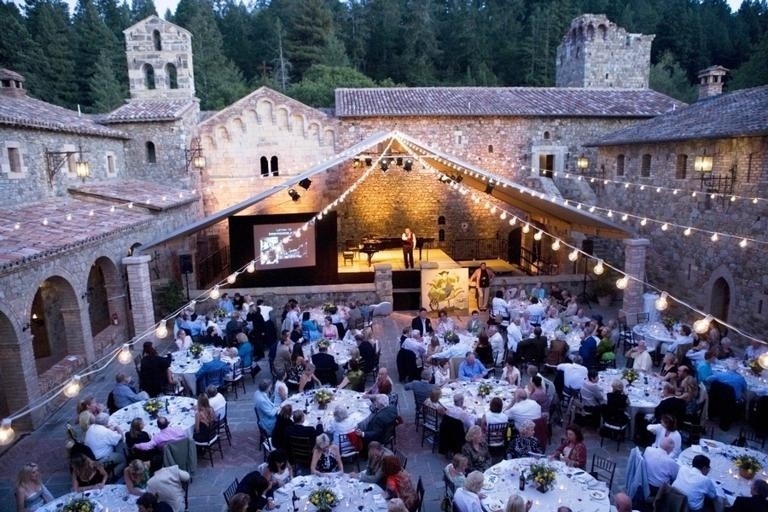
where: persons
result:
[401,228,417,269]
[14,261,768,510]
[261,241,277,264]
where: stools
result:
[343,250,358,266]
[349,247,360,260]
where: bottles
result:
[519,471,526,491]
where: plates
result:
[481,457,518,511]
[523,453,606,512]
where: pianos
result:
[358,234,435,267]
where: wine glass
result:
[269,475,384,512]
[43,392,195,511]
[723,354,767,388]
[285,387,370,429]
[167,311,237,370]
[417,293,692,411]
[301,302,360,357]
[691,444,766,492]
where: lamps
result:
[577,152,589,174]
[484,183,494,195]
[76,152,89,183]
[694,148,715,179]
[194,150,205,175]
[437,174,463,185]
[353,159,412,172]
[288,189,300,201]
